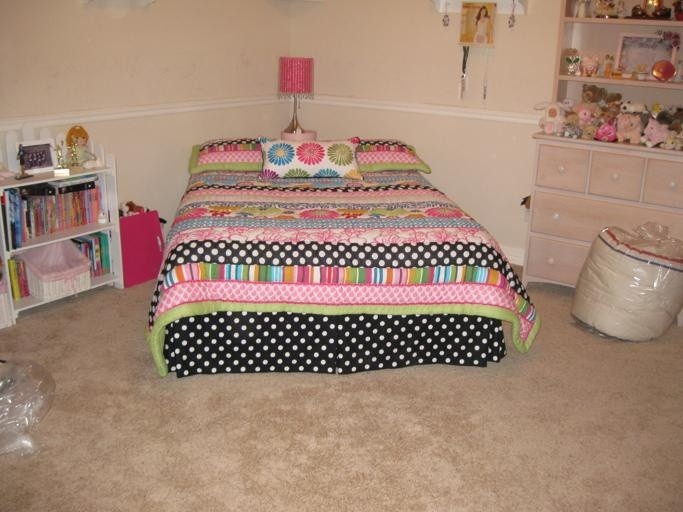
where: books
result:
[0,175,110,302]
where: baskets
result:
[21,241,92,303]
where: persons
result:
[473,6,491,43]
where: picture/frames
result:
[457,1,496,48]
[16,139,60,177]
[614,32,679,82]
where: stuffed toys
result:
[65,126,96,165]
[533,84,683,151]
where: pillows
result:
[355,138,430,174]
[188,138,262,176]
[257,137,363,181]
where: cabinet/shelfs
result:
[519,130,683,291]
[1,165,124,333]
[550,0,683,107]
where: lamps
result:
[277,56,317,143]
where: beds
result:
[145,172,540,380]
